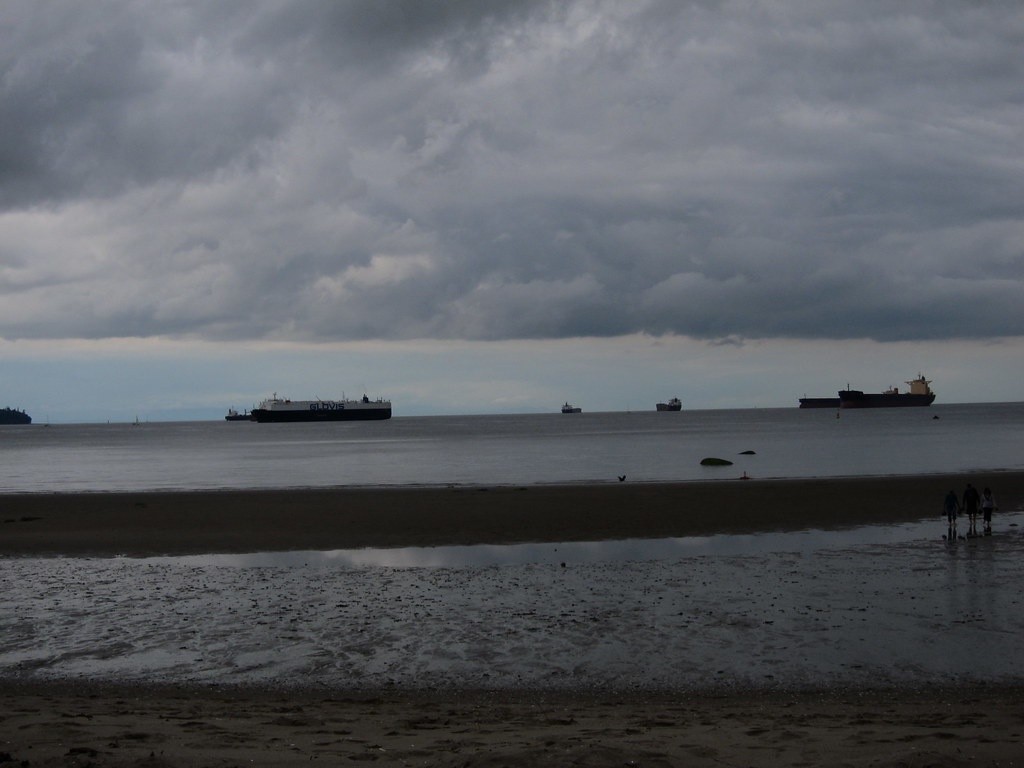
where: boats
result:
[561,402,582,413]
[656,396,681,412]
[224,406,256,421]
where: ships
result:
[249,393,392,423]
[798,372,936,409]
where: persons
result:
[942,489,961,524]
[963,483,981,524]
[980,487,999,525]
